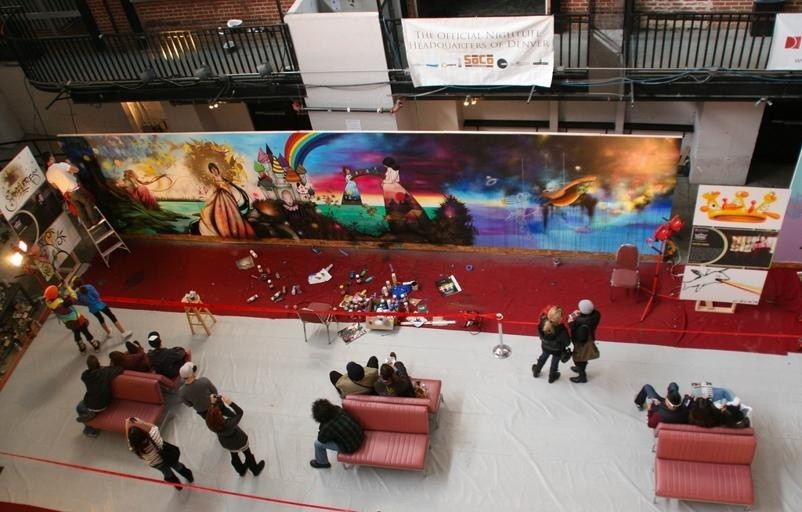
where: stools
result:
[180,294,216,336]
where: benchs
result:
[654,423,755,437]
[83,374,171,440]
[340,377,444,430]
[121,362,184,395]
[336,400,430,472]
[653,432,756,509]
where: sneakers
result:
[308,459,331,470]
[105,326,115,340]
[119,329,134,340]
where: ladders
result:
[79,206,131,268]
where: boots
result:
[163,474,183,491]
[178,465,194,482]
[532,359,544,380]
[245,454,266,477]
[569,363,584,385]
[76,338,87,357]
[547,366,561,384]
[231,458,248,477]
[88,338,101,352]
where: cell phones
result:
[570,312,576,317]
[214,394,222,398]
[130,417,137,424]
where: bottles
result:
[246,247,428,324]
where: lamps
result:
[195,66,213,78]
[462,95,477,107]
[138,69,155,82]
[257,63,273,76]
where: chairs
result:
[296,302,338,344]
[609,244,641,303]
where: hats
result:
[179,363,200,379]
[577,299,595,316]
[344,362,365,382]
[146,331,161,349]
[666,382,681,409]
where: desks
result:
[0,283,36,326]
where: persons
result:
[205,393,265,477]
[124,415,194,491]
[75,354,124,439]
[633,382,744,428]
[176,361,235,421]
[309,351,416,469]
[567,298,601,383]
[34,193,56,229]
[73,277,133,338]
[531,303,571,383]
[146,331,186,378]
[44,280,101,355]
[42,150,100,228]
